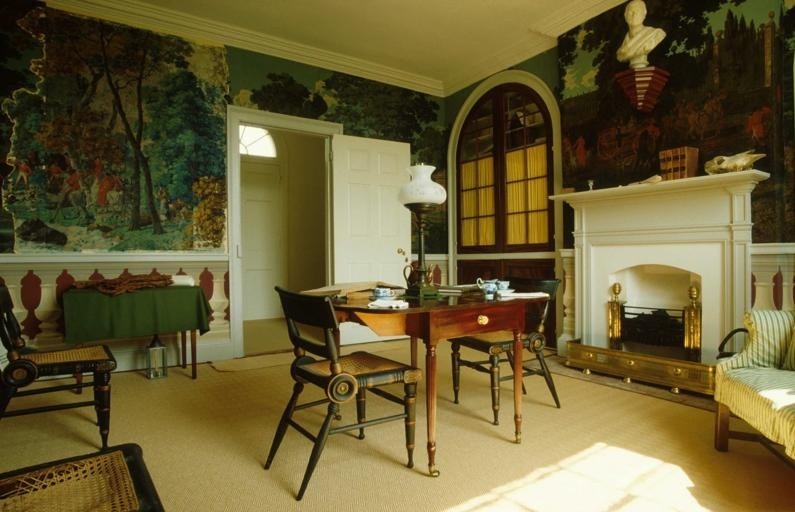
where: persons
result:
[617,2,667,70]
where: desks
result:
[62,284,214,395]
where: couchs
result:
[714,310,794,472]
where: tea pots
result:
[476,278,500,300]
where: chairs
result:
[0,279,117,451]
[0,442,164,512]
[264,287,422,501]
[447,277,561,426]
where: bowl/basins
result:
[496,280,510,289]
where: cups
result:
[373,288,394,296]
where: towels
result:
[369,299,407,309]
[498,290,550,300]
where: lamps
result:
[399,165,447,300]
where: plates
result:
[497,288,515,294]
